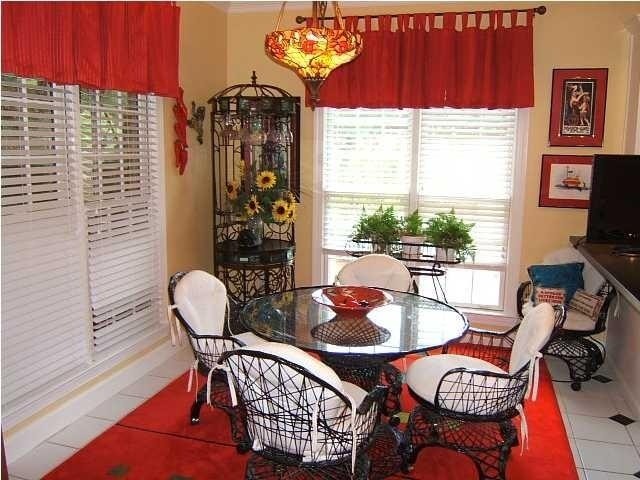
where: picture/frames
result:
[548,66,609,148]
[539,155,595,210]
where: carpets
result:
[43,344,579,480]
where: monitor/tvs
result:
[584,153,640,258]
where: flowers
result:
[226,162,296,224]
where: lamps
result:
[264,2,363,111]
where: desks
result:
[240,285,470,393]
[350,240,461,303]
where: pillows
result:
[569,289,605,321]
[535,287,566,308]
[528,262,585,301]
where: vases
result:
[245,215,265,247]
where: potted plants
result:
[399,208,427,259]
[426,209,477,263]
[352,204,400,255]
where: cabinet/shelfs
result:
[572,236,640,424]
[207,69,295,336]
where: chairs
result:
[167,268,271,454]
[210,343,403,480]
[334,252,412,294]
[398,302,567,480]
[517,279,618,392]
